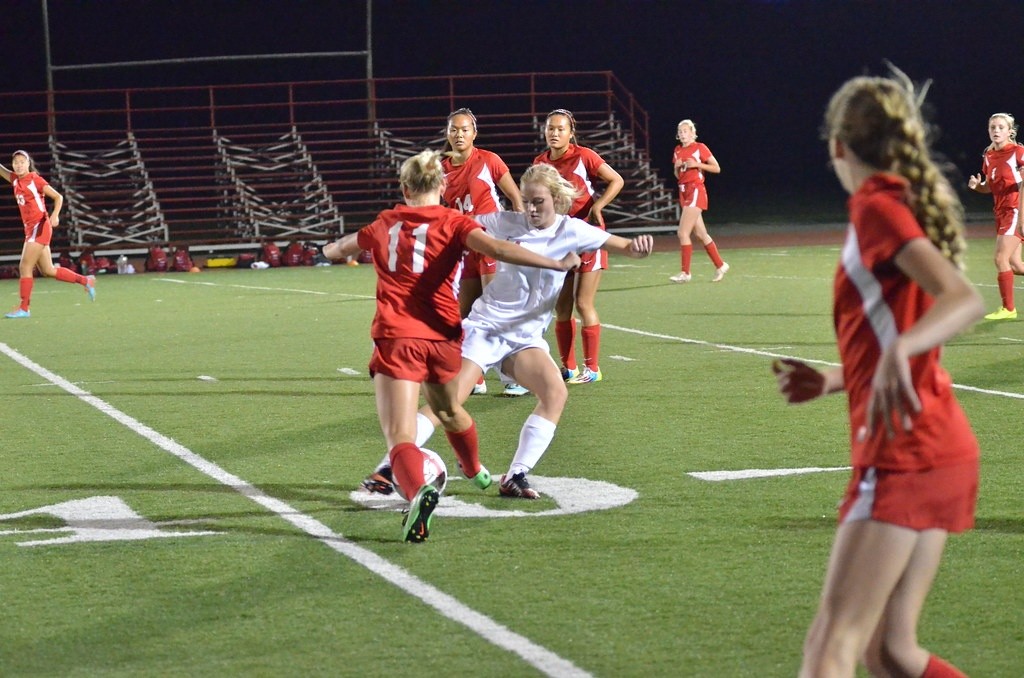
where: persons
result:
[321,148,581,542]
[671,118,729,284]
[350,164,654,511]
[772,75,987,678]
[967,113,1024,319]
[0,150,96,318]
[434,107,529,396]
[534,109,625,385]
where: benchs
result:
[0,118,687,260]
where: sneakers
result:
[502,381,530,397]
[983,306,1018,320]
[6,307,31,318]
[713,262,730,281]
[470,380,487,395]
[669,269,691,281]
[84,274,96,301]
[499,471,541,498]
[559,364,580,380]
[361,466,394,494]
[457,457,491,489]
[566,364,604,385]
[403,483,439,544]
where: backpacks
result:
[0,253,115,279]
[235,239,321,269]
[173,248,194,272]
[143,245,167,272]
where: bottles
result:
[116,254,128,274]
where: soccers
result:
[392,447,449,504]
